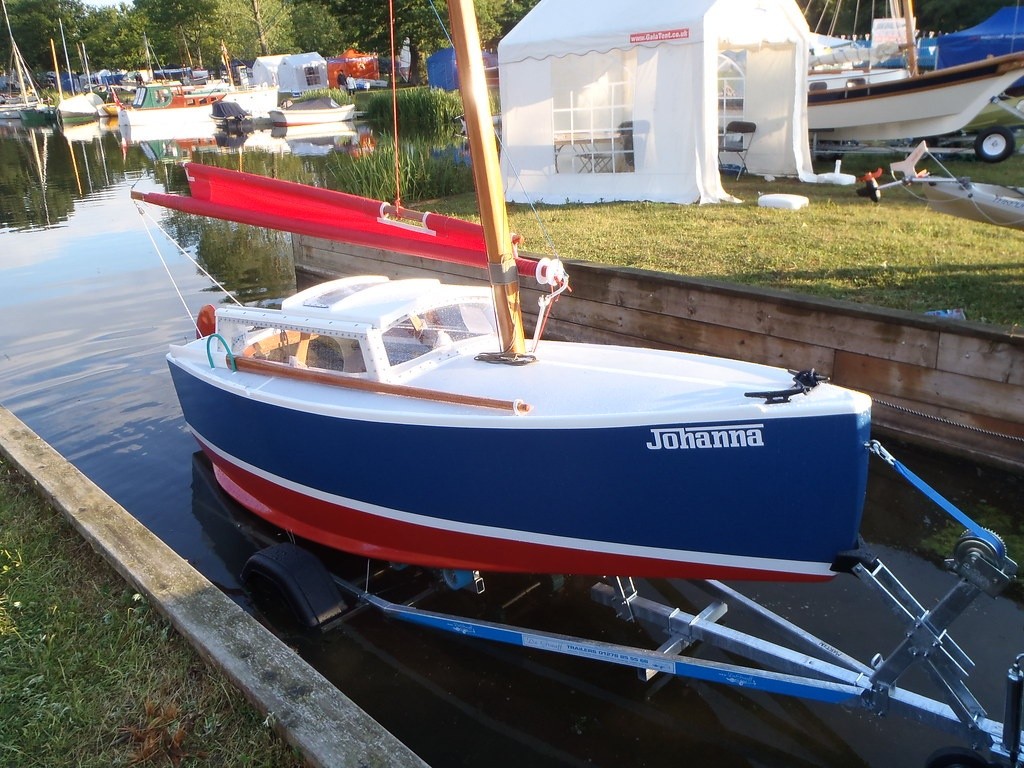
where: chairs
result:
[571,144,612,173]
[717,121,757,181]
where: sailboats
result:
[28,123,244,231]
[0,0,279,125]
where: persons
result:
[337,69,347,91]
[346,74,357,95]
[135,73,138,81]
[139,74,142,85]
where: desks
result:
[554,134,621,172]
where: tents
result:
[328,48,379,89]
[937,5,1024,91]
[427,45,489,91]
[251,51,328,91]
[497,0,854,209]
[0,65,190,94]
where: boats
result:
[122,0,1024,768]
[208,94,277,129]
[268,95,355,129]
[626,0,1024,219]
